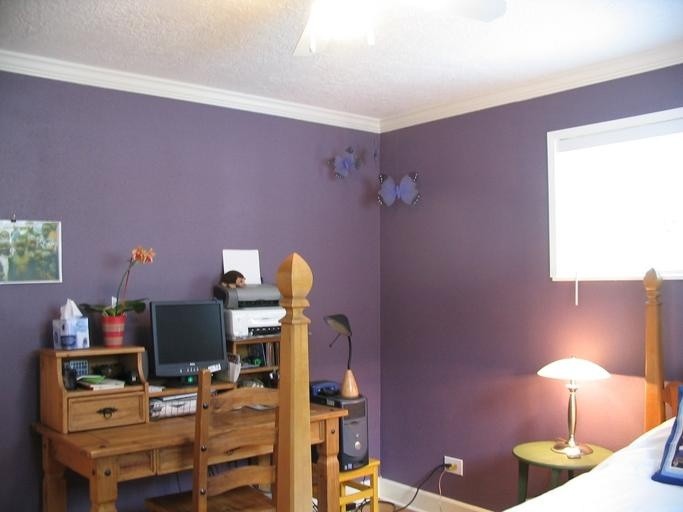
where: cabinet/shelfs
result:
[38,344,153,434]
[146,375,236,423]
[224,337,280,410]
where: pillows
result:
[654,380,683,418]
[649,384,682,490]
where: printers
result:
[214,247,281,308]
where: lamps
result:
[535,353,612,461]
[321,310,363,400]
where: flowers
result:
[76,242,157,318]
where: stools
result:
[312,457,381,512]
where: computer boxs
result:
[315,394,369,471]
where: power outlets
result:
[444,455,464,477]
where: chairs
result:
[140,364,281,512]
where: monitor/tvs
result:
[149,299,229,387]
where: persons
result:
[0,223,59,281]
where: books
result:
[76,375,125,391]
[249,342,280,367]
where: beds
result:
[268,249,683,512]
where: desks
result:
[30,399,348,512]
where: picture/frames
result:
[0,220,63,284]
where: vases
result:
[97,313,126,350]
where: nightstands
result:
[510,438,616,505]
